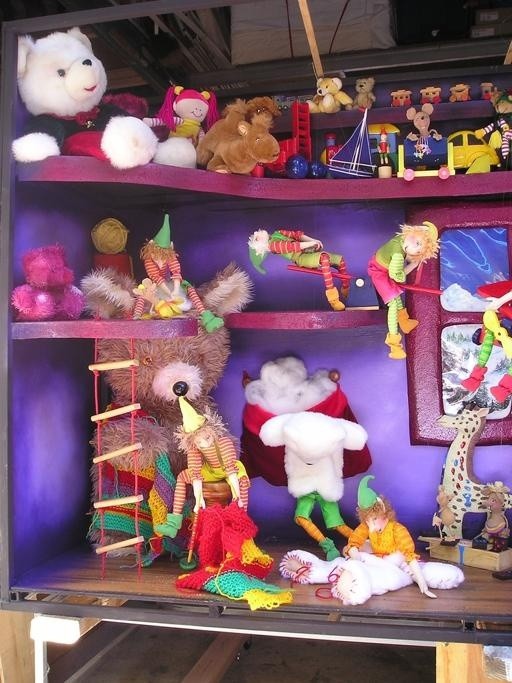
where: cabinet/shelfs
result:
[0,64,512,647]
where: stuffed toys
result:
[15,26,512,607]
